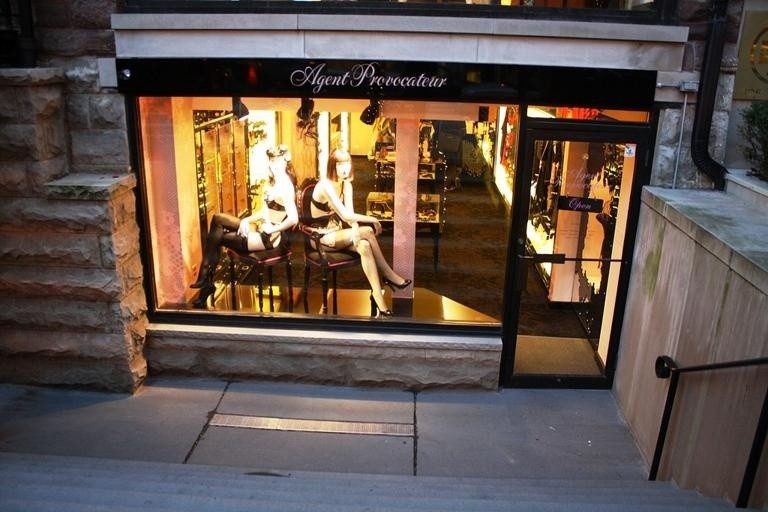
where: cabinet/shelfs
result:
[368,137,446,265]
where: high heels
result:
[382,274,412,293]
[192,284,216,306]
[370,289,392,316]
[189,263,214,288]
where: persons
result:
[189,144,300,307]
[308,148,412,318]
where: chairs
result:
[226,184,377,318]
